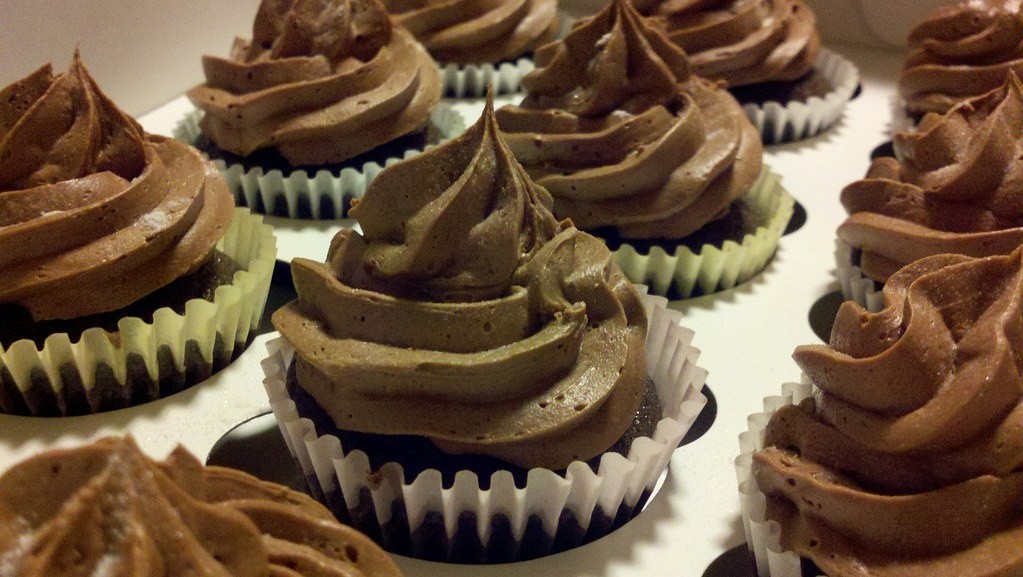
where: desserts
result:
[0,0,1023,577]
